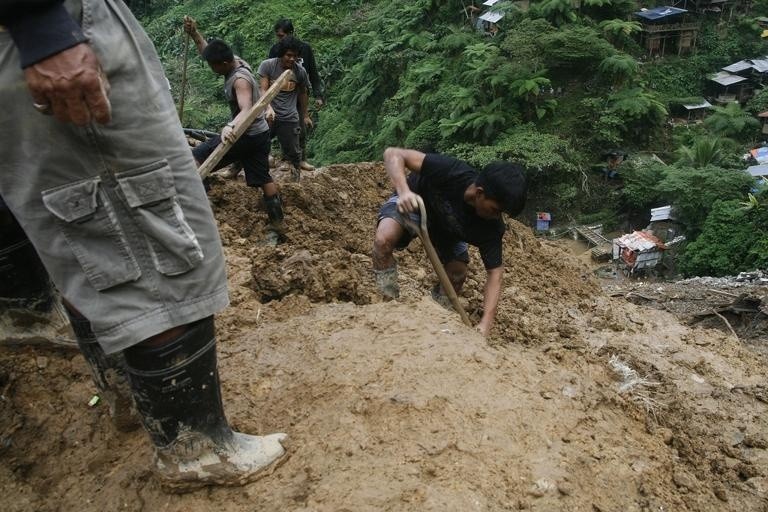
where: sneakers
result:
[280,160,316,172]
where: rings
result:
[33,102,51,113]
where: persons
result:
[370,146,531,339]
[2,0,298,497]
[270,20,326,171]
[181,13,287,248]
[219,35,315,185]
[1,197,81,350]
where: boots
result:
[122,315,295,493]
[220,159,244,180]
[374,261,400,304]
[61,310,142,433]
[260,190,288,243]
[431,285,457,313]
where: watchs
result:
[225,121,237,129]
